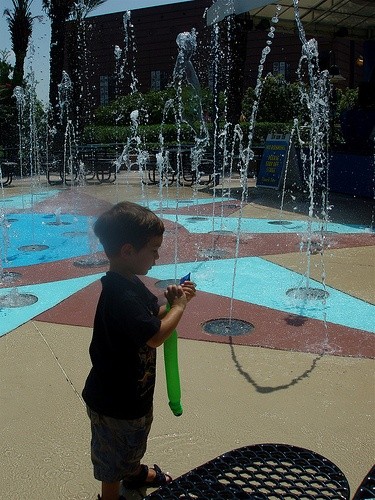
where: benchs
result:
[43,144,117,186]
[0,146,20,187]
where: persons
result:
[81,202,197,500]
[199,114,218,144]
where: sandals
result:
[121,462,173,489]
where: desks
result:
[131,146,218,186]
